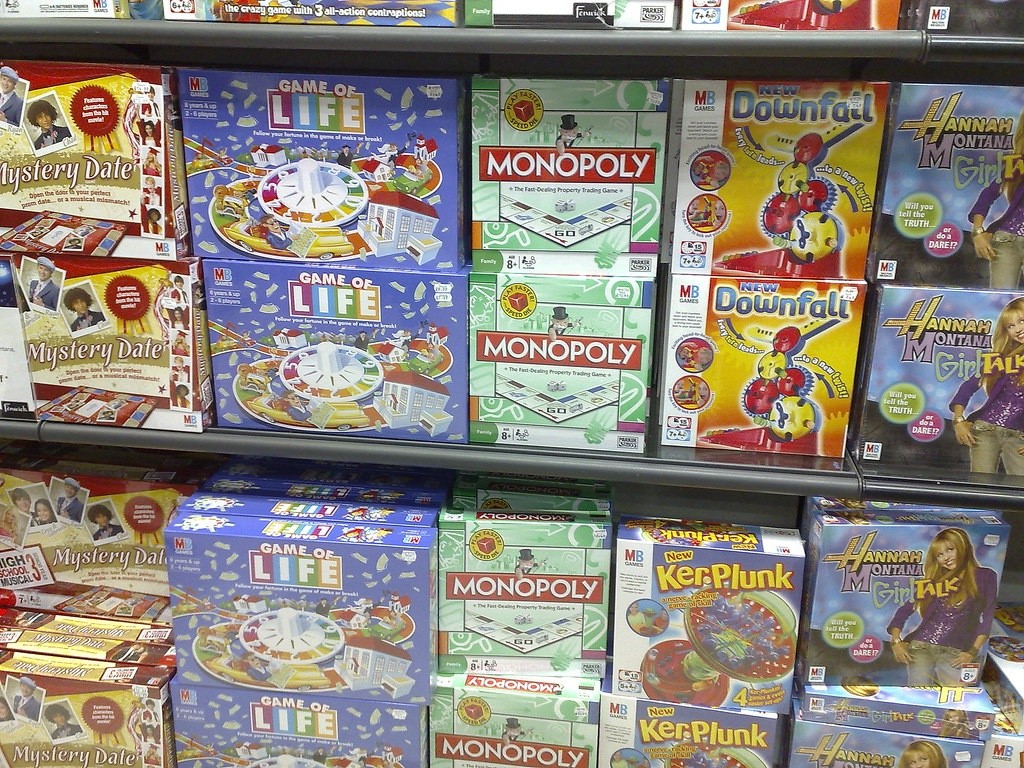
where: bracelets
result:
[969,647,980,657]
[969,226,985,238]
[952,416,965,426]
[889,637,902,647]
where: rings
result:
[961,436,965,438]
[988,246,991,250]
[980,250,983,252]
[898,656,902,658]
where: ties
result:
[182,292,186,303]
[154,104,158,116]
[153,713,158,722]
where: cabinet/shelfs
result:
[0,17,1024,512]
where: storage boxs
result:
[0,60,191,262]
[200,258,470,444]
[669,77,890,280]
[848,284,1024,476]
[12,255,216,434]
[613,0,676,29]
[464,0,614,28]
[0,253,38,422]
[162,0,464,27]
[658,273,868,461]
[175,63,472,272]
[898,0,1024,38]
[681,0,729,30]
[468,271,655,453]
[0,446,1024,768]
[866,81,1024,291]
[0,0,131,19]
[470,69,672,278]
[727,0,900,31]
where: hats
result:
[21,677,35,689]
[150,744,159,749]
[178,330,188,337]
[37,257,55,271]
[65,478,81,489]
[149,147,160,155]
[1,67,19,82]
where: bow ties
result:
[59,726,65,731]
[43,131,51,137]
[79,314,86,321]
[100,526,106,532]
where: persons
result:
[0,677,83,740]
[27,100,70,149]
[131,697,165,768]
[136,84,166,236]
[0,65,24,128]
[29,225,88,251]
[29,256,60,311]
[170,275,191,409]
[61,287,105,330]
[833,113,1024,767]
[0,473,124,544]
